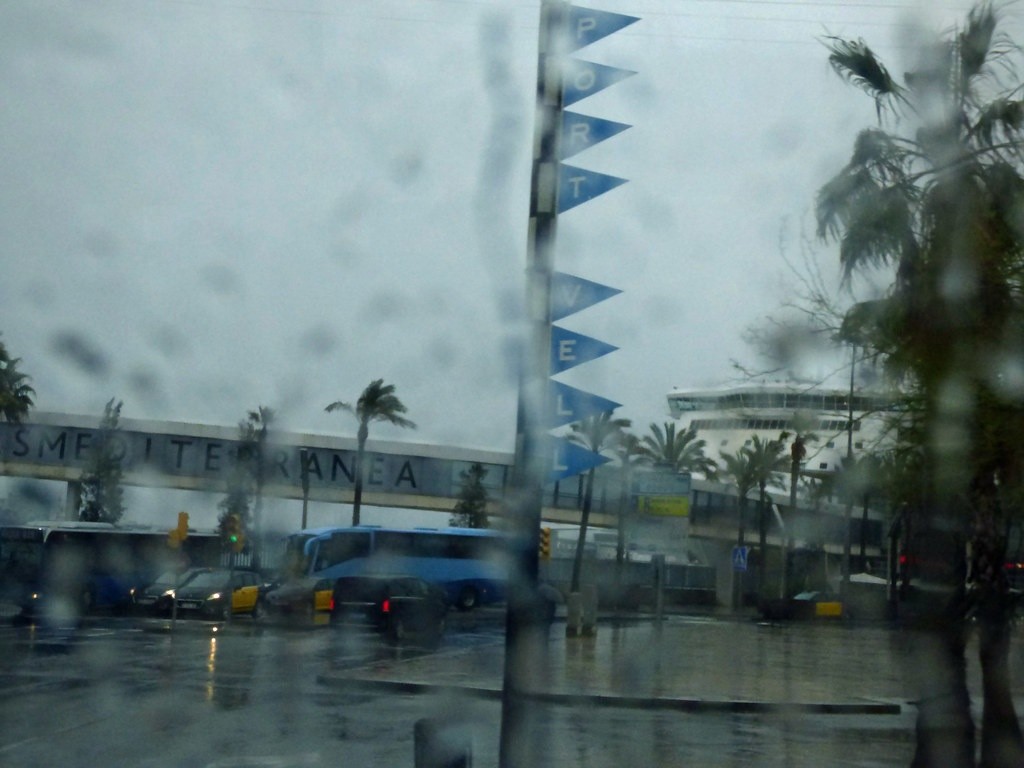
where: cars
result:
[126,557,448,633]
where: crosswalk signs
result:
[731,545,750,572]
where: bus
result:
[277,520,537,610]
[0,513,224,615]
